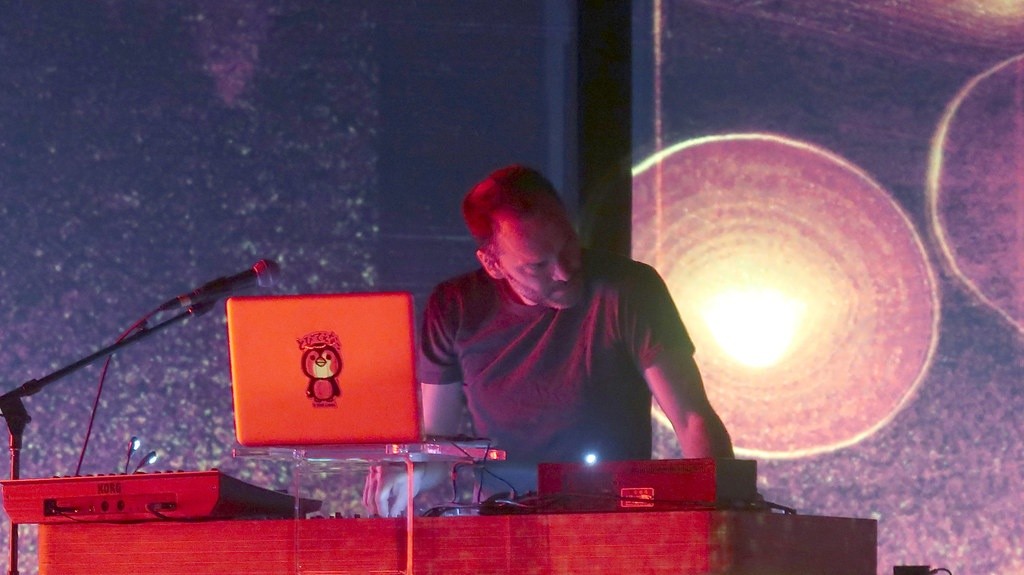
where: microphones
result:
[160,259,280,311]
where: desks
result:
[38,513,878,575]
[232,444,507,575]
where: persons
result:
[364,163,736,517]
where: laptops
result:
[224,292,507,462]
